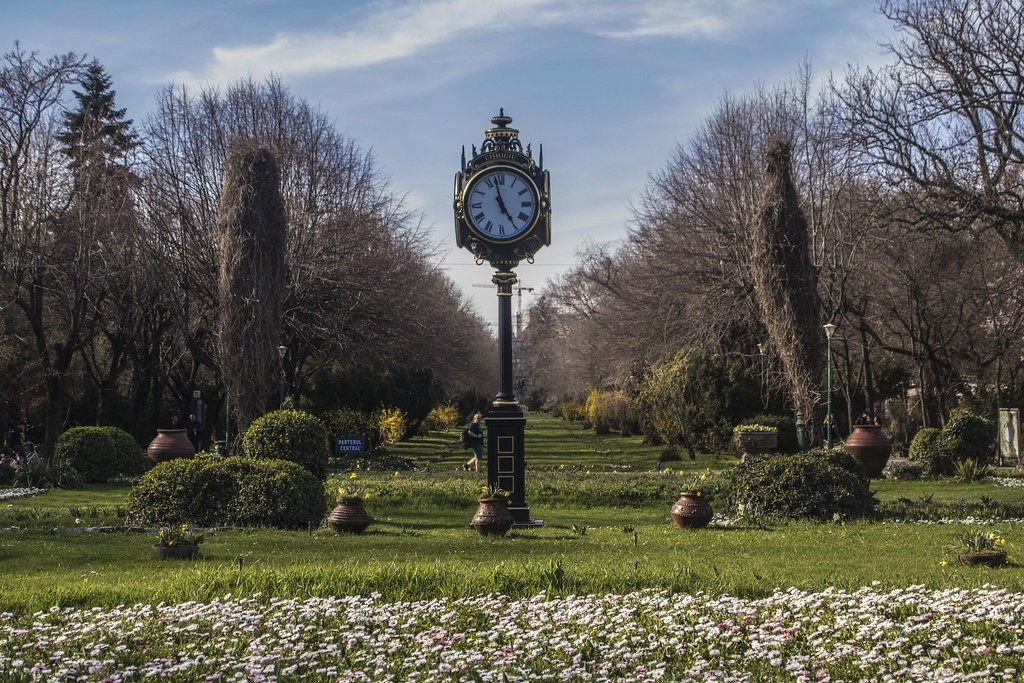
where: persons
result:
[187,415,204,453]
[855,410,882,426]
[821,414,838,448]
[463,413,484,472]
[3,418,34,459]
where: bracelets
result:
[477,435,478,438]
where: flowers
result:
[479,481,513,505]
[666,466,712,497]
[955,527,1007,551]
[154,522,203,547]
[337,471,373,501]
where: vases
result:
[152,542,199,560]
[471,497,514,536]
[327,498,371,535]
[671,492,713,529]
[956,552,1007,566]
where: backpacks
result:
[460,426,473,450]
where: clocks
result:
[461,164,541,243]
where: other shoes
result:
[463,464,468,471]
